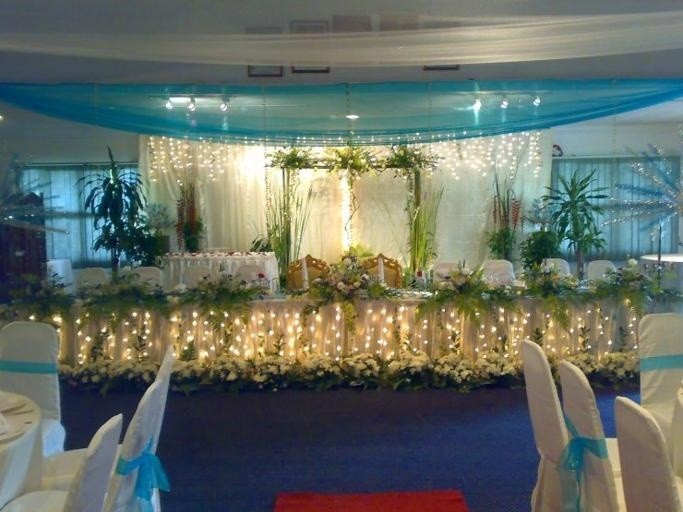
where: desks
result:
[47,257,73,295]
[162,249,279,294]
[640,252,683,293]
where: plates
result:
[0,416,27,440]
[0,396,25,412]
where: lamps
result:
[162,87,233,113]
[460,91,542,114]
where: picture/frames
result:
[241,14,460,78]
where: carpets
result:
[270,489,468,512]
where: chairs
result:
[289,253,617,287]
[2,318,183,512]
[75,266,163,294]
[517,302,682,509]
